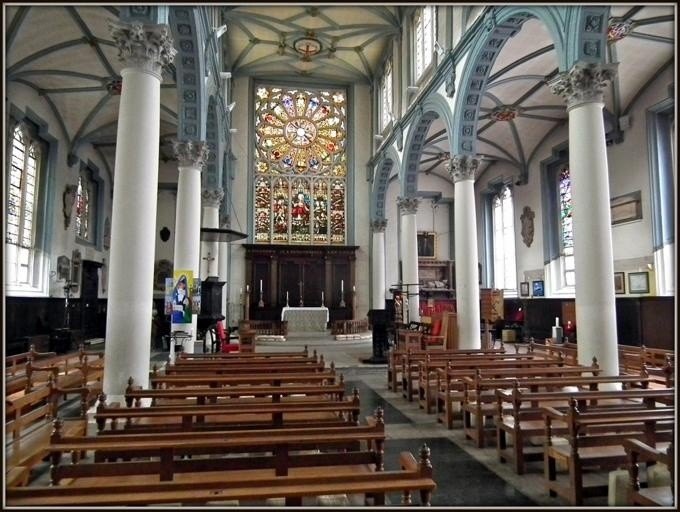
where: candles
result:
[260,279,262,291]
[287,291,288,299]
[321,292,324,300]
[341,280,344,292]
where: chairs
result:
[7,342,437,506]
[386,339,675,507]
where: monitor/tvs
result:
[417,232,437,259]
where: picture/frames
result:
[613,271,650,294]
[520,279,544,297]
